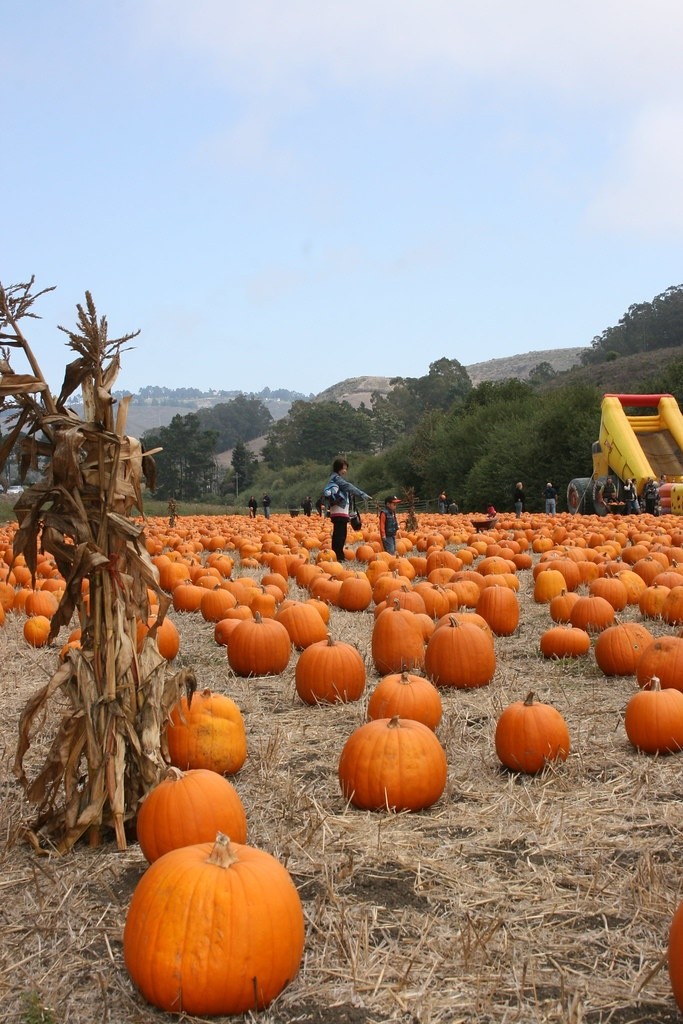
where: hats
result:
[385,496,402,503]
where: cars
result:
[0,484,5,494]
[6,486,24,494]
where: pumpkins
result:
[0,495,683,1015]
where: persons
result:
[263,492,271,519]
[439,491,459,515]
[328,459,373,564]
[514,482,525,518]
[599,474,666,515]
[248,495,257,519]
[379,495,402,555]
[304,494,324,517]
[487,503,496,518]
[542,483,558,516]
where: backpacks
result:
[323,480,345,507]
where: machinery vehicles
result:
[566,390,683,515]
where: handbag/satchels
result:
[351,516,361,530]
